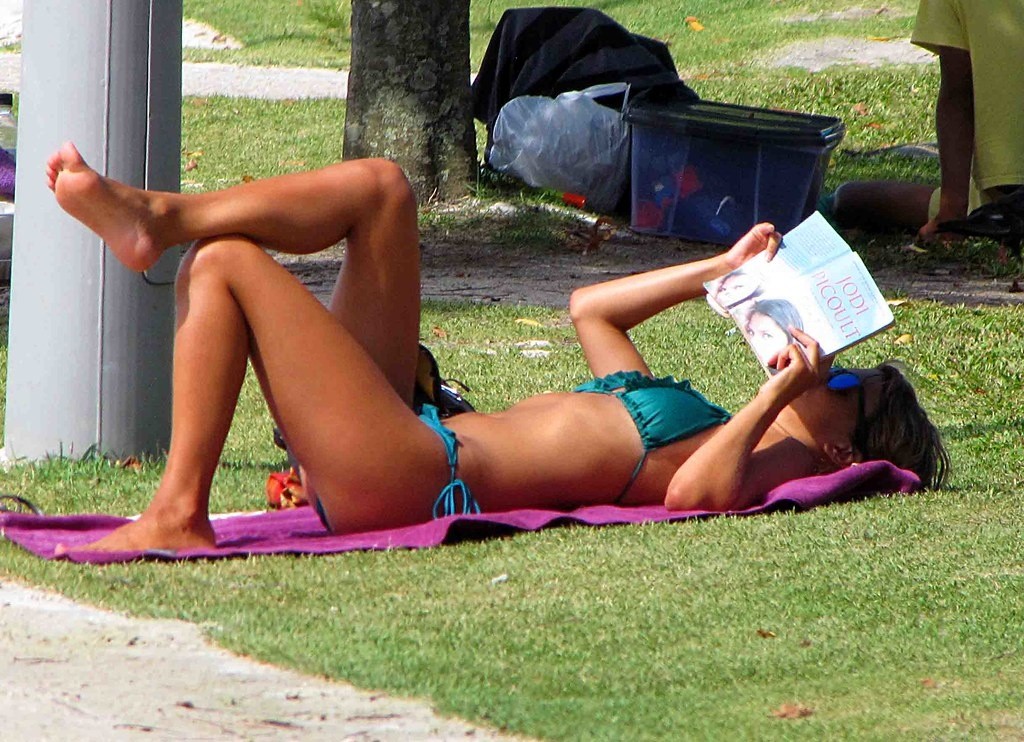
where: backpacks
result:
[413,344,475,419]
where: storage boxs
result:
[623,97,846,245]
[816,71,817,74]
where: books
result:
[703,210,897,379]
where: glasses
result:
[825,363,869,442]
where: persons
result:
[830,0,1023,250]
[714,269,804,368]
[44,141,946,554]
